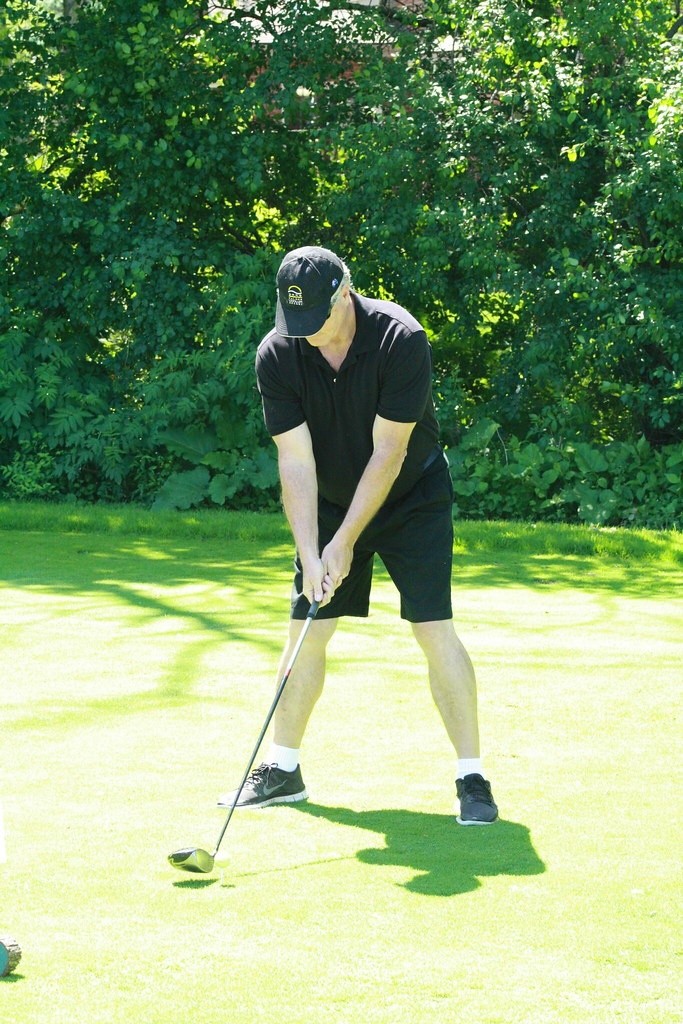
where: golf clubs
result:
[165,599,321,876]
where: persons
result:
[218,246,498,826]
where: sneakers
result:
[217,761,309,809]
[455,773,499,825]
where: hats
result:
[275,246,344,338]
